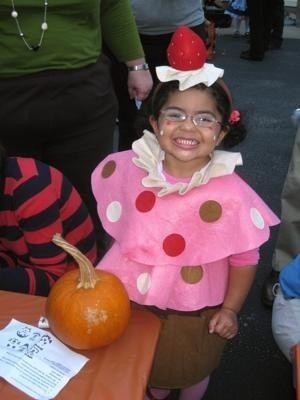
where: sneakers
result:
[240,41,281,61]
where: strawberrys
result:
[166,26,207,71]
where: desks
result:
[0,290,161,400]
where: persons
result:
[90,78,282,400]
[261,115,299,305]
[111,0,284,151]
[1,1,154,242]
[0,146,98,296]
[271,254,300,362]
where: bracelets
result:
[221,306,238,315]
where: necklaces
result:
[11,0,49,51]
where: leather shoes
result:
[262,270,279,305]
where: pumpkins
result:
[44,235,131,350]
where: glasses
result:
[160,109,225,130]
